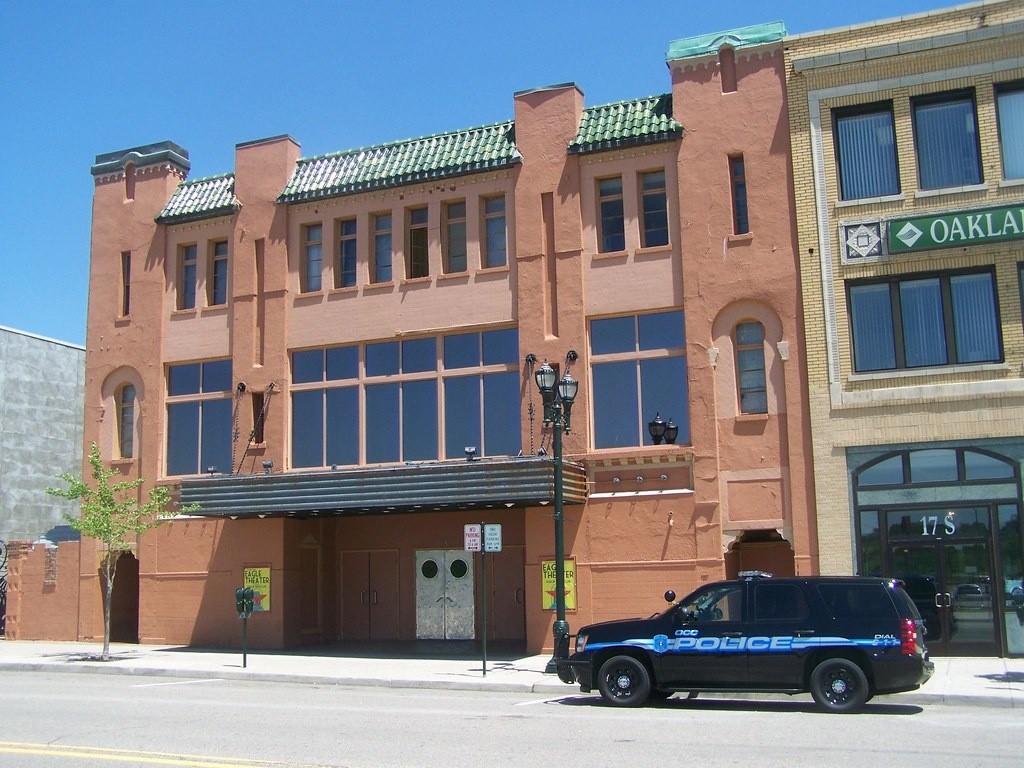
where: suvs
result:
[564,570,938,713]
[903,574,957,642]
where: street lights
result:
[903,549,909,573]
[532,358,579,673]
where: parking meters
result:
[235,587,253,666]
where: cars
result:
[972,574,1024,624]
[950,583,984,611]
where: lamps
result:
[464,446,478,461]
[206,465,217,477]
[537,446,547,457]
[263,460,274,475]
